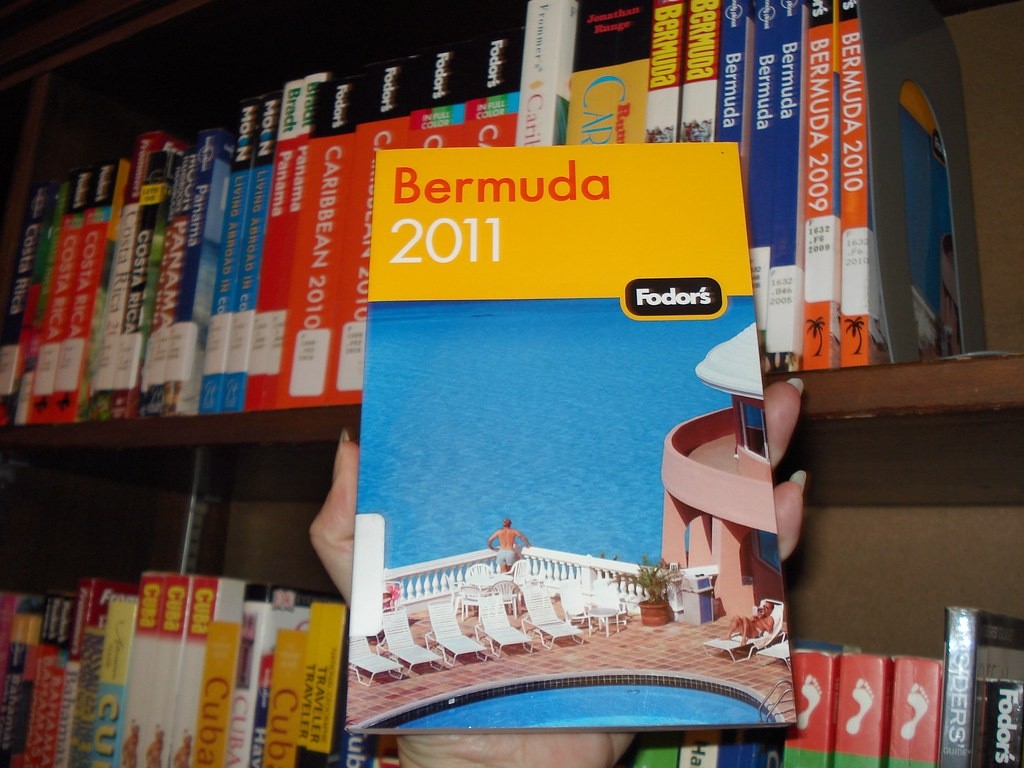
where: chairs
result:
[349,558,627,687]
[702,598,792,672]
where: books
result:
[345,142,798,735]
[0,570,1024,768]
[0,0,962,424]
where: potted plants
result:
[607,554,684,627]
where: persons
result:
[718,601,774,647]
[310,377,808,768]
[487,519,530,572]
